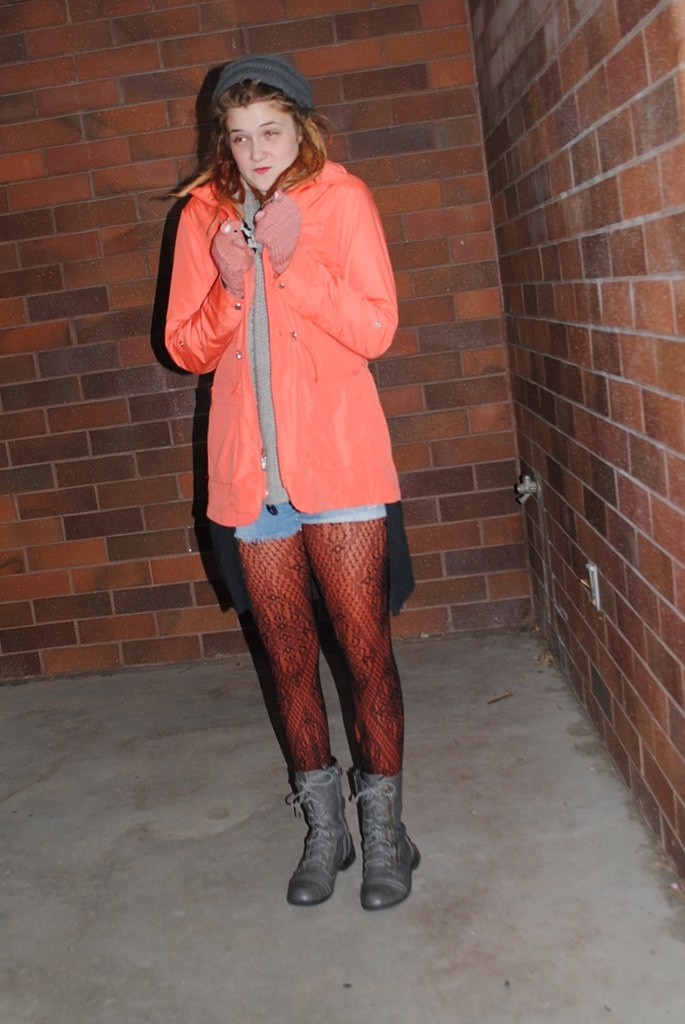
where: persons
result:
[163,52,421,909]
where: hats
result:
[210,54,321,110]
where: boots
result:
[285,757,365,902]
[352,769,421,911]
